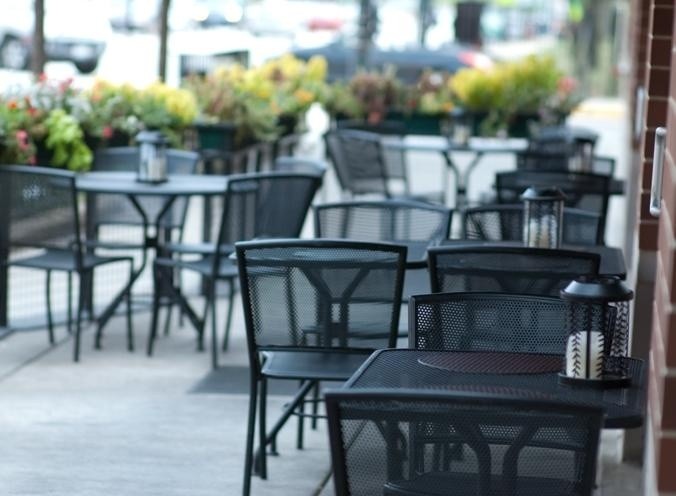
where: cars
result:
[0,23,102,75]
[279,36,495,77]
